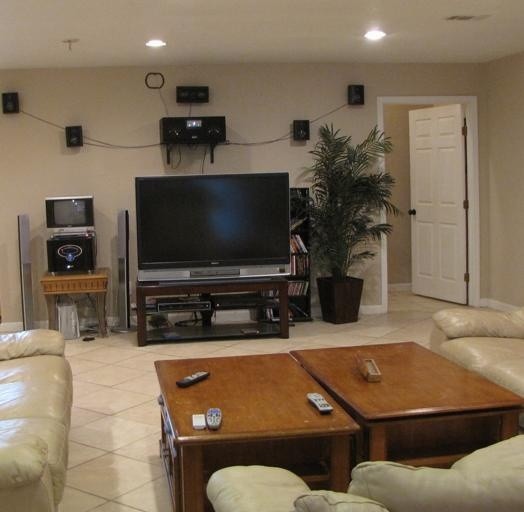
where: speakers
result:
[293,120,310,140]
[18,214,33,331]
[65,126,83,147]
[112,208,137,334]
[348,85,364,105]
[1,92,20,114]
[176,86,209,103]
[160,116,226,144]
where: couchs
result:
[206,433,524,512]
[430,303,523,392]
[0,327,75,512]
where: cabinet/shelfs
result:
[290,187,313,322]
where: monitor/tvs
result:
[43,194,96,238]
[135,172,291,282]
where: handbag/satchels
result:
[54,294,80,341]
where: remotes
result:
[176,371,211,388]
[307,392,334,414]
[205,407,223,430]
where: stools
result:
[39,268,111,339]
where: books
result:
[287,281,308,295]
[288,302,311,321]
[290,233,309,276]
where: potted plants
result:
[291,120,403,326]
